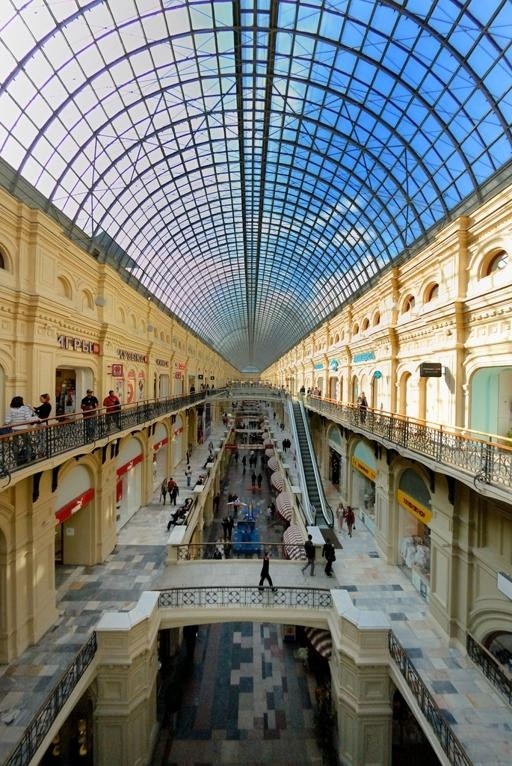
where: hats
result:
[86,388,94,393]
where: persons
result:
[235,452,239,463]
[322,539,336,577]
[336,503,346,534]
[253,455,258,468]
[251,472,256,487]
[258,551,278,592]
[242,456,246,466]
[282,439,287,452]
[300,535,316,577]
[286,439,291,448]
[200,383,215,391]
[237,420,260,444]
[185,466,192,487]
[266,383,289,394]
[103,390,123,431]
[204,456,213,468]
[213,516,234,559]
[165,498,193,530]
[191,475,203,491]
[358,392,368,423]
[159,477,179,506]
[345,506,355,539]
[81,389,98,437]
[209,441,213,454]
[272,410,276,420]
[249,455,254,468]
[257,473,263,488]
[232,494,239,512]
[29,393,52,455]
[224,414,228,427]
[299,385,321,396]
[271,503,275,520]
[215,492,220,512]
[190,384,195,394]
[280,423,285,432]
[4,396,40,466]
[186,448,191,464]
[266,506,272,520]
[228,493,232,510]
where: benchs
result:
[182,495,199,527]
[201,470,211,486]
